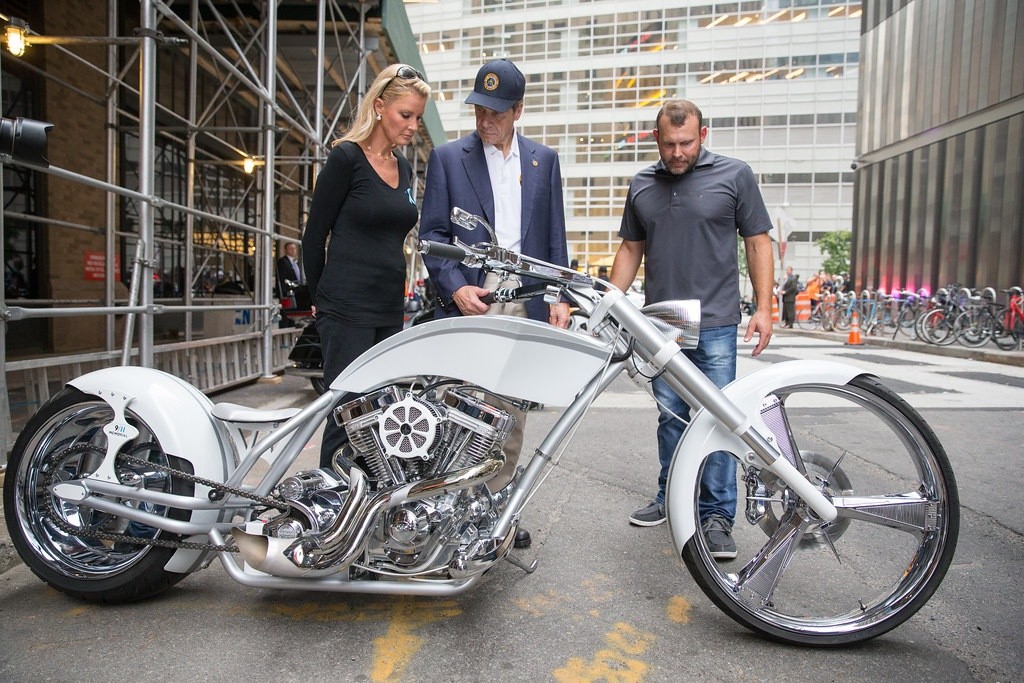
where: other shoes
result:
[780,323,792,329]
[514,526,532,548]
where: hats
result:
[571,259,578,266]
[598,266,607,273]
[464,58,526,113]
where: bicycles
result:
[796,287,1024,353]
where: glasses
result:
[378,66,425,98]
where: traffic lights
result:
[3,19,30,58]
[0,118,56,169]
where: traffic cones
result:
[844,308,866,346]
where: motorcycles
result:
[1,205,965,651]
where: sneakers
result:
[700,513,737,558]
[629,500,667,526]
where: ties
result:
[291,259,300,282]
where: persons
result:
[277,241,303,296]
[590,265,610,297]
[419,57,571,549]
[602,100,776,560]
[568,259,579,272]
[804,269,851,308]
[781,265,797,329]
[774,274,803,322]
[299,63,431,471]
